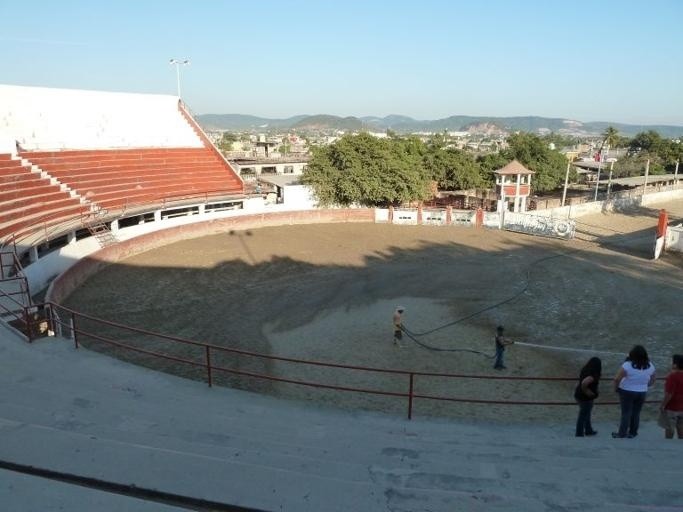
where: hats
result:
[396,305,405,312]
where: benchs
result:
[0,144,246,512]
[247,390,682,511]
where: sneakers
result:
[611,432,638,439]
[575,430,598,437]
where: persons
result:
[610,344,656,439]
[493,325,514,371]
[391,306,405,345]
[658,353,683,440]
[573,356,602,437]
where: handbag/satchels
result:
[574,385,599,401]
